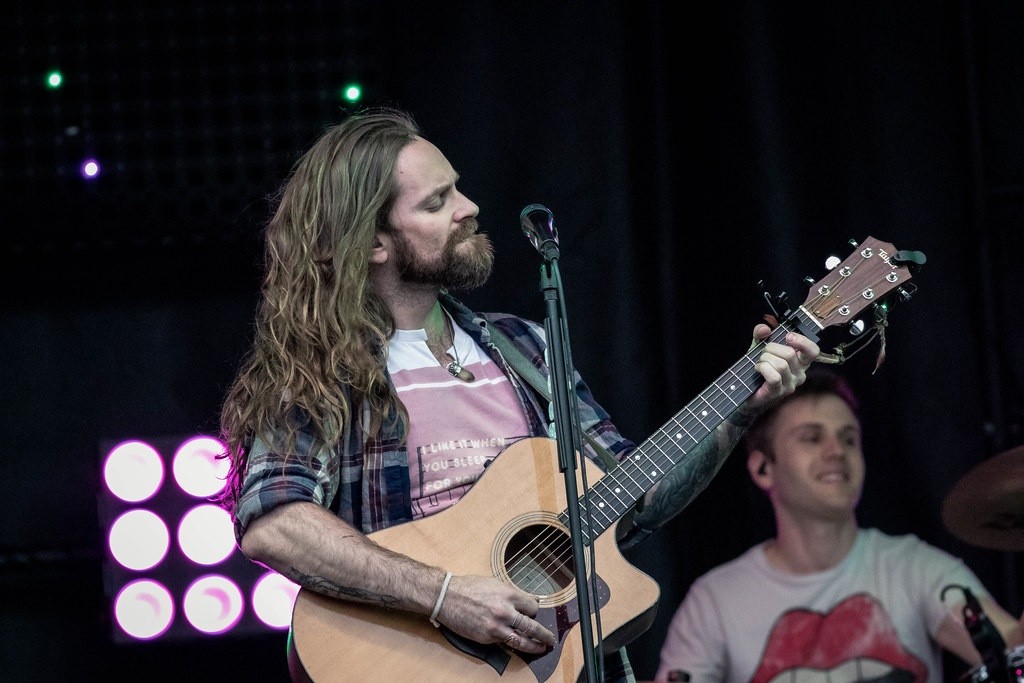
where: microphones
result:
[519,203,559,260]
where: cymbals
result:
[940,445,1023,554]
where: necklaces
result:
[429,315,476,384]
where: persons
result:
[218,108,823,683]
[655,369,1024,683]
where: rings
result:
[503,634,522,652]
[511,611,522,628]
[517,615,531,634]
[514,613,524,628]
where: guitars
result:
[286,234,929,681]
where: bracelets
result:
[428,571,453,629]
